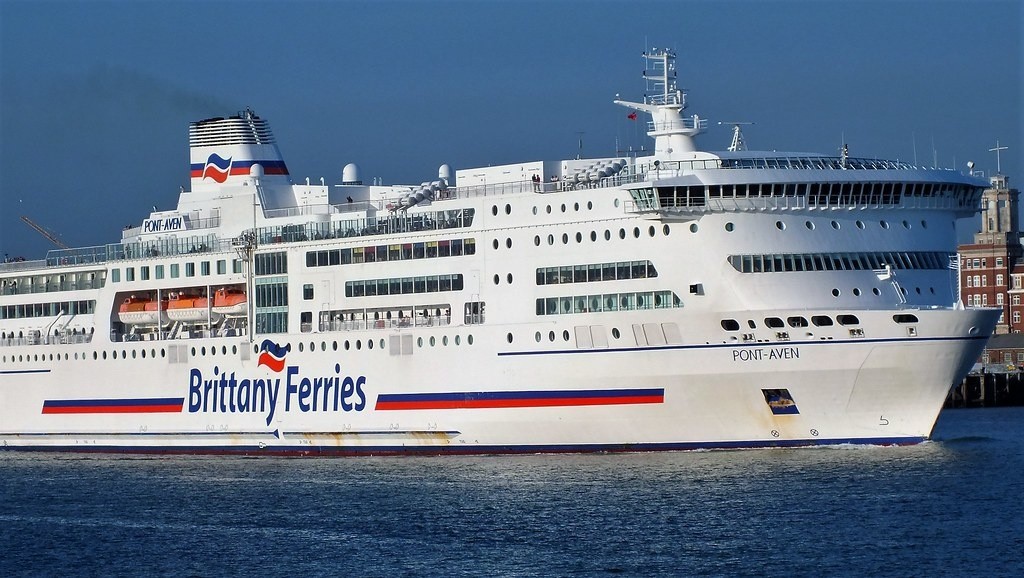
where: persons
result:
[346,196,353,203]
[532,173,540,191]
[125,225,132,229]
[3,279,16,294]
[144,244,157,256]
[297,213,463,241]
[3,253,25,263]
[550,175,559,190]
[60,257,68,265]
[189,244,212,253]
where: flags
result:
[628,111,637,120]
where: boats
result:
[211,287,248,315]
[166,292,214,321]
[116,295,169,324]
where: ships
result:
[0,33,1005,454]
